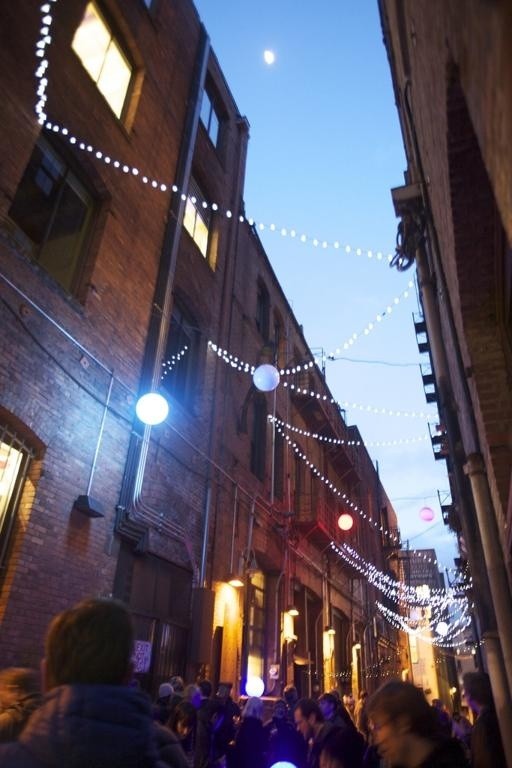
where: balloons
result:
[254,363,281,394]
[419,507,436,522]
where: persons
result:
[2,597,505,768]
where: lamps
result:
[286,605,299,616]
[325,626,336,634]
[74,495,106,517]
[227,576,244,586]
[353,641,361,649]
